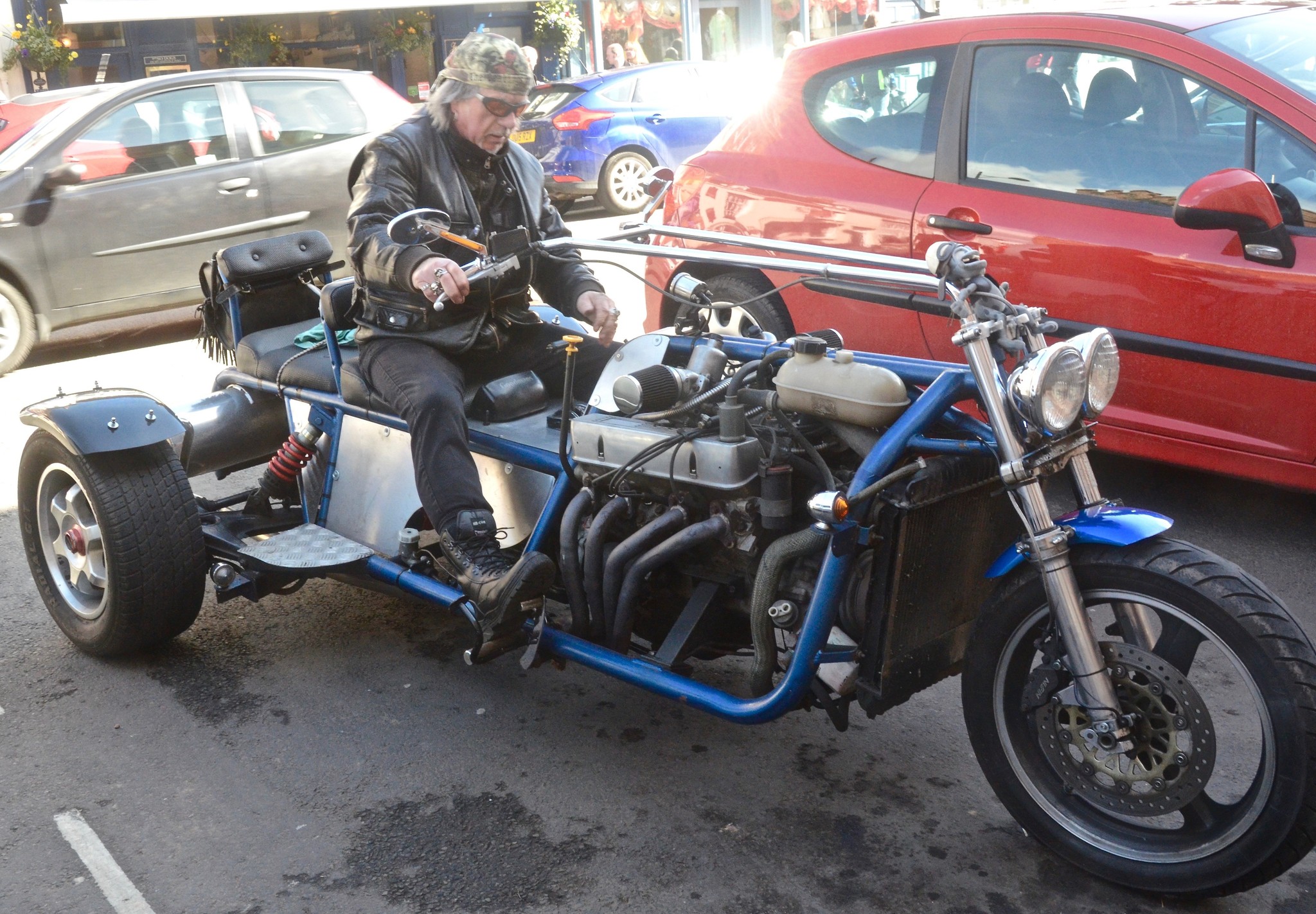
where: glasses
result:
[469,89,530,119]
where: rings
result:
[431,281,443,296]
[421,283,430,291]
[611,309,620,317]
[434,268,449,278]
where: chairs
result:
[977,67,1154,186]
[828,112,929,149]
[317,274,484,416]
[216,228,363,391]
[168,142,197,168]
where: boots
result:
[439,507,556,638]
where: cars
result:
[510,58,776,217]
[0,84,286,187]
[0,65,415,386]
[641,1,1316,500]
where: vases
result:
[27,60,57,72]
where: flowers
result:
[1,6,78,75]
[213,18,290,66]
[525,0,586,77]
[371,9,437,64]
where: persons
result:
[783,31,804,58]
[606,43,634,68]
[848,10,896,116]
[625,41,650,64]
[663,39,682,61]
[347,26,620,643]
[1026,52,1082,110]
[522,46,538,71]
[120,118,177,172]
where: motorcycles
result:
[16,163,1316,902]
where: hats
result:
[439,29,533,95]
